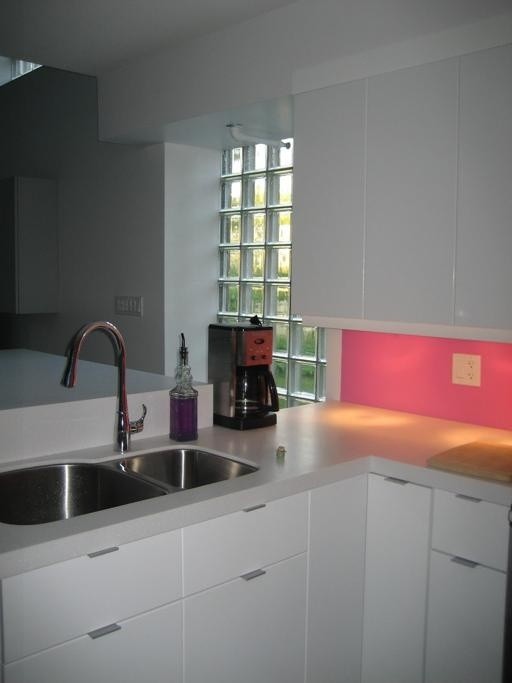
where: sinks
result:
[0,458,173,530]
[117,443,260,491]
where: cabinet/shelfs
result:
[185,490,309,682]
[422,487,511,682]
[291,78,369,331]
[308,473,367,683]
[360,473,430,683]
[363,55,460,339]
[2,526,186,683]
[454,41,511,343]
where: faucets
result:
[62,319,148,451]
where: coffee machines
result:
[207,315,279,432]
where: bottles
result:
[169,331,200,443]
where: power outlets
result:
[450,351,481,388]
